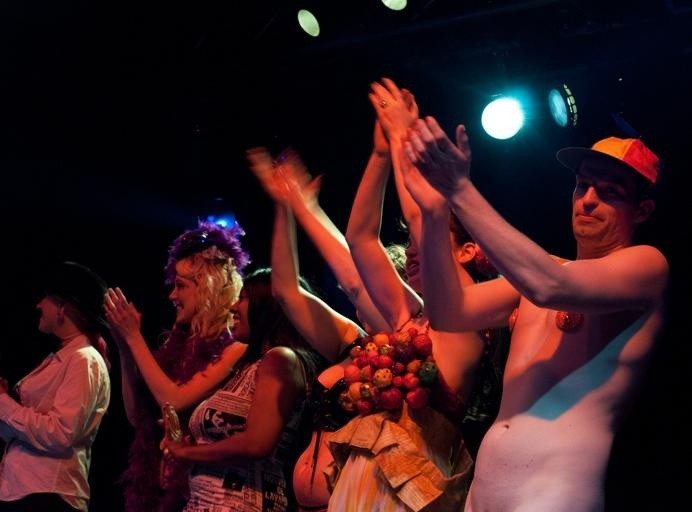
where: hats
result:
[558,139,660,197]
[22,258,102,318]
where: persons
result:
[2,262,111,510]
[101,221,322,512]
[328,78,508,511]
[248,147,409,510]
[398,116,668,510]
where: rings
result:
[381,100,387,107]
